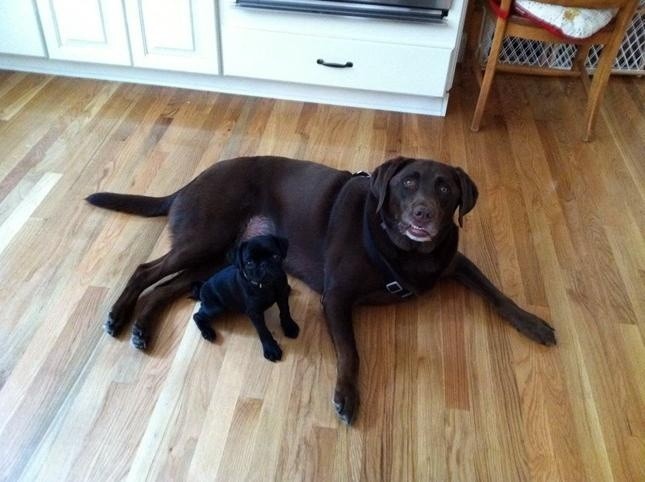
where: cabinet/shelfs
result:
[0,2,48,76]
[219,0,469,116]
[35,0,220,94]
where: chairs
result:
[459,0,639,143]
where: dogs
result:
[84,156,556,427]
[181,234,300,362]
